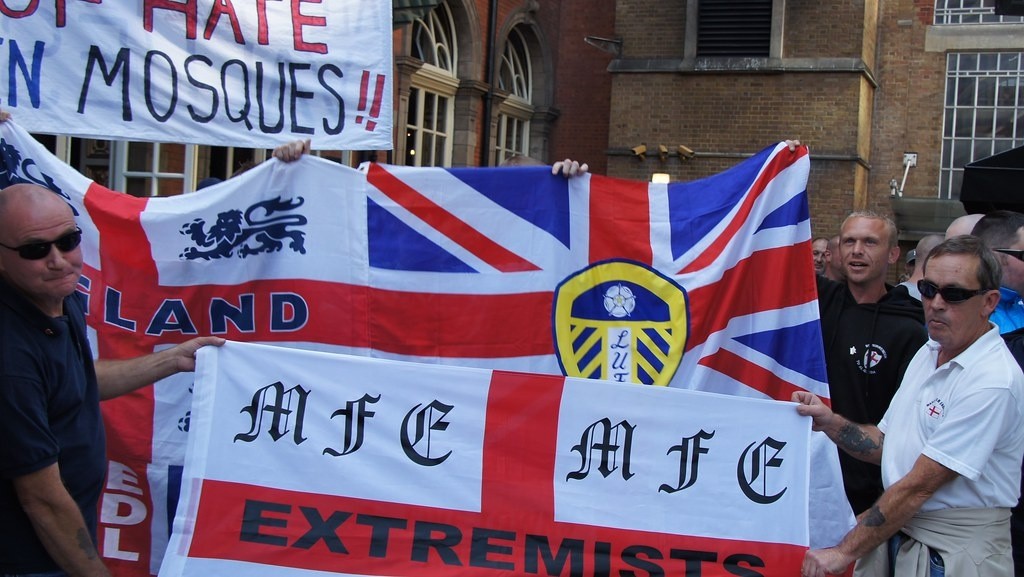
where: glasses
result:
[0,226,83,260]
[917,280,990,304]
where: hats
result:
[904,248,916,263]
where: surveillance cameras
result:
[677,145,695,158]
[658,145,668,157]
[630,145,646,155]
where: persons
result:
[791,234,1024,577]
[271,139,588,177]
[813,235,846,283]
[785,138,928,515]
[0,184,225,577]
[898,213,1024,577]
